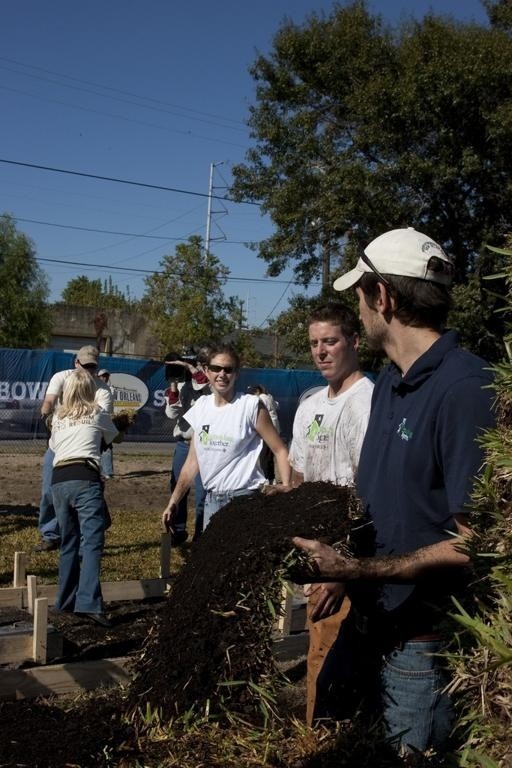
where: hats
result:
[97,368,110,377]
[75,344,100,367]
[330,227,455,295]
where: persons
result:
[33,346,113,554]
[100,368,115,480]
[165,345,215,545]
[51,374,126,625]
[161,344,291,542]
[284,303,378,735]
[277,226,510,768]
[245,381,283,436]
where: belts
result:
[348,608,442,644]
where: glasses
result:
[205,363,235,375]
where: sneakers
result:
[75,609,113,628]
